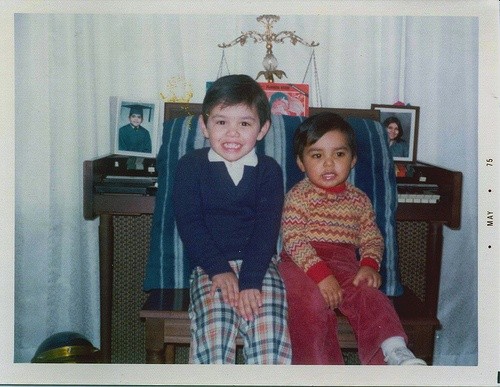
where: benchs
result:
[140,286,441,366]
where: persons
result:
[117,104,152,154]
[174,73,293,365]
[383,115,409,158]
[274,110,428,365]
[268,91,297,117]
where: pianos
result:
[82,154,463,367]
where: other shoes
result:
[385,345,428,366]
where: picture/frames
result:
[257,82,310,119]
[114,99,160,158]
[371,103,420,163]
